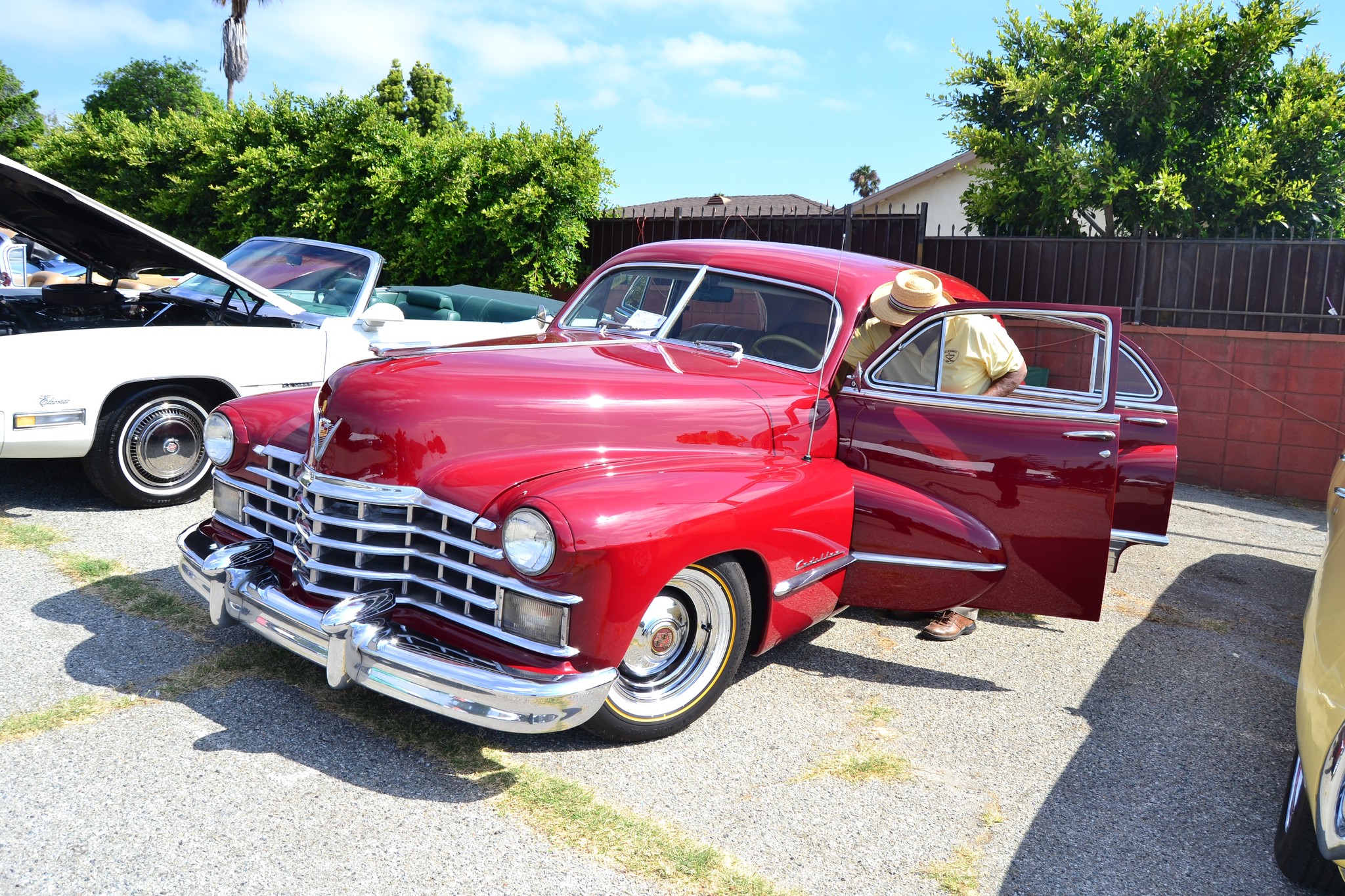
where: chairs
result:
[320,277,384,308]
[778,319,835,349]
[677,323,774,357]
[395,288,462,321]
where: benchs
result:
[424,288,548,321]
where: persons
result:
[837,268,1027,642]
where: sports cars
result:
[0,154,612,510]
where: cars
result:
[174,237,1181,750]
[1272,449,1344,891]
[1,227,192,298]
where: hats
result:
[870,268,957,327]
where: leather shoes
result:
[920,610,975,640]
